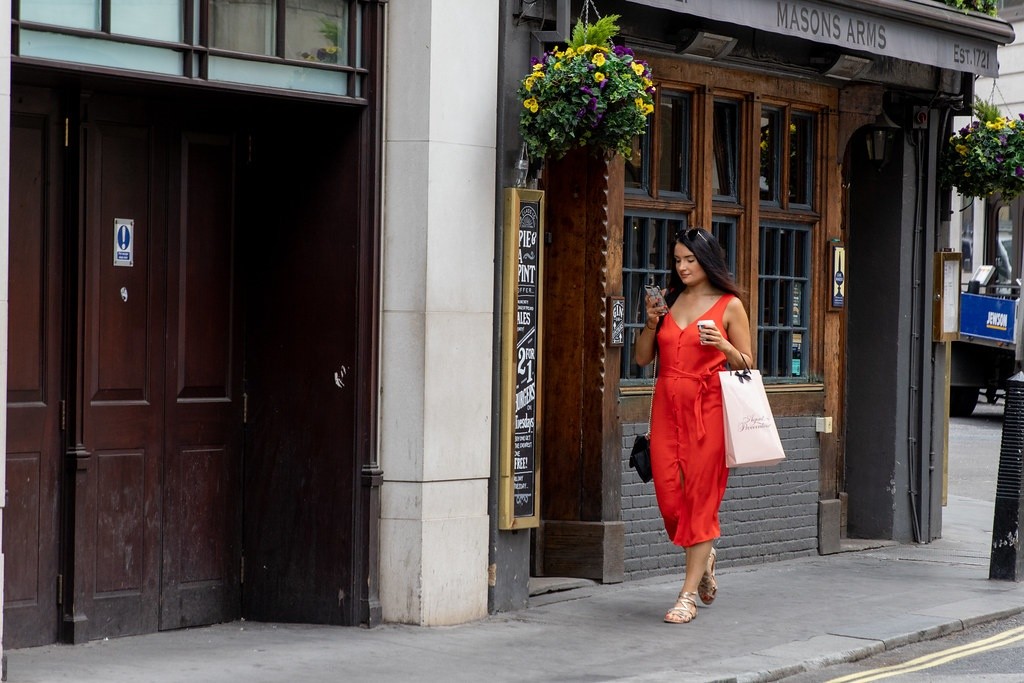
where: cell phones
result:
[644,284,668,314]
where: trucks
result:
[949,281,1024,418]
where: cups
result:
[697,320,715,345]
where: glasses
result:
[675,228,707,242]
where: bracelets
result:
[646,324,656,330]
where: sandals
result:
[664,592,698,623]
[697,547,718,605]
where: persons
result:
[635,227,753,624]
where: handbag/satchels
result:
[718,352,786,469]
[628,434,653,484]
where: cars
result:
[962,231,1013,281]
